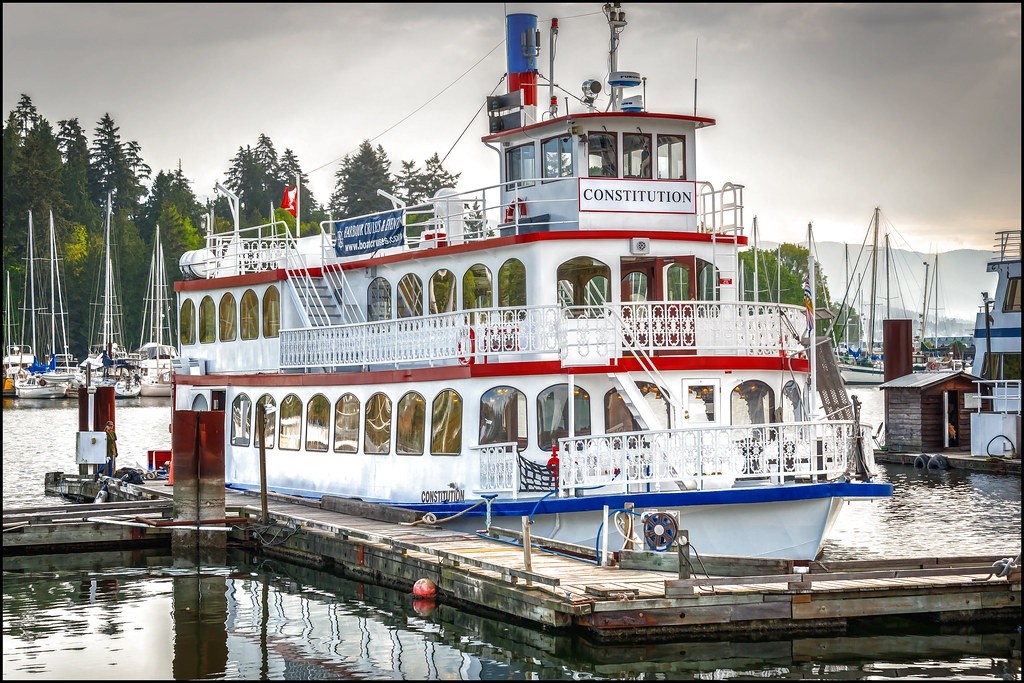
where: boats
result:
[170,0,899,578]
[971,229,1024,411]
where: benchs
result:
[279,253,308,268]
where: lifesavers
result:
[927,454,948,469]
[457,325,477,365]
[506,197,527,223]
[777,334,805,358]
[913,454,929,470]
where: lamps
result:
[573,388,589,399]
[734,382,768,401]
[689,386,713,399]
[450,391,461,403]
[641,383,663,400]
[487,387,510,402]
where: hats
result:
[107,421,113,426]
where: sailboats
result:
[830,206,975,386]
[0,192,178,399]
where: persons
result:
[95,421,118,478]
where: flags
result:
[281,184,298,217]
